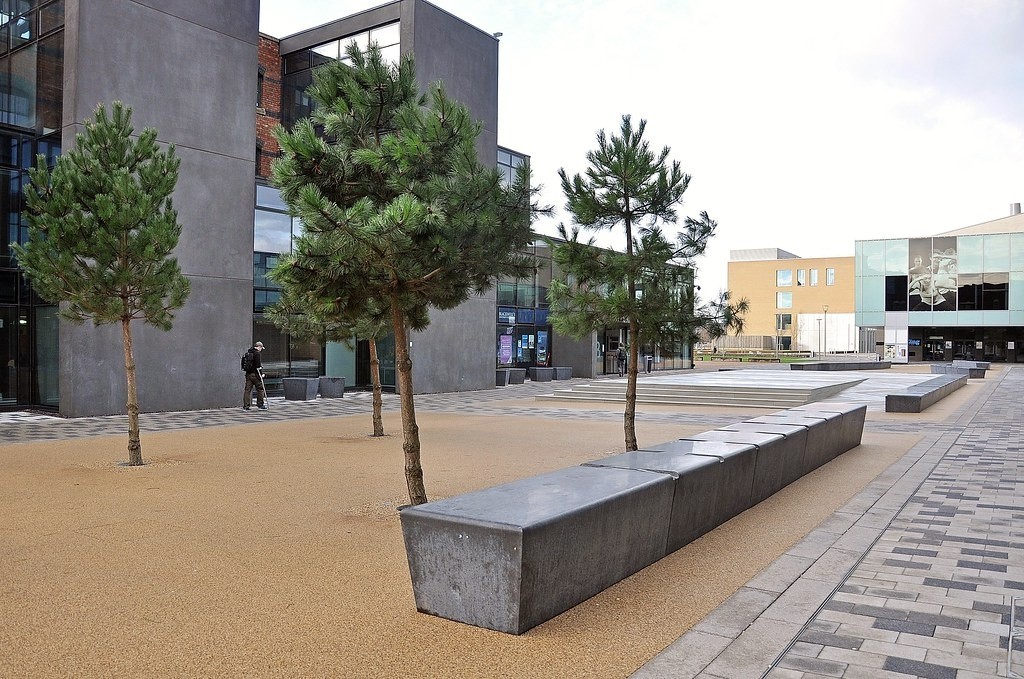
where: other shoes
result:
[619,373,623,377]
[243,408,252,413]
[257,405,269,411]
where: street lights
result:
[773,313,781,359]
[816,318,823,361]
[822,304,830,359]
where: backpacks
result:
[240,350,259,372]
[617,348,627,362]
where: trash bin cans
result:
[644,356,652,374]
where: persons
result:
[242,342,268,411]
[909,249,956,311]
[616,342,628,377]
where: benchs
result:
[400,401,866,636]
[885,375,967,413]
[790,361,891,371]
[953,360,991,369]
[932,365,986,379]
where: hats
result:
[255,341,266,349]
